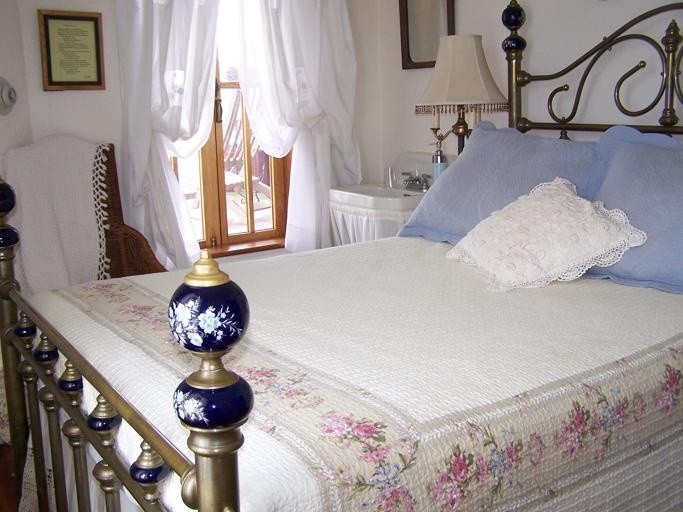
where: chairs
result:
[2,134,169,295]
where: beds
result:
[2,3,683,512]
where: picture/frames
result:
[39,8,107,94]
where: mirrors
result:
[399,0,454,72]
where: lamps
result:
[415,32,510,170]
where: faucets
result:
[403,175,423,189]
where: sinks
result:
[329,184,420,210]
